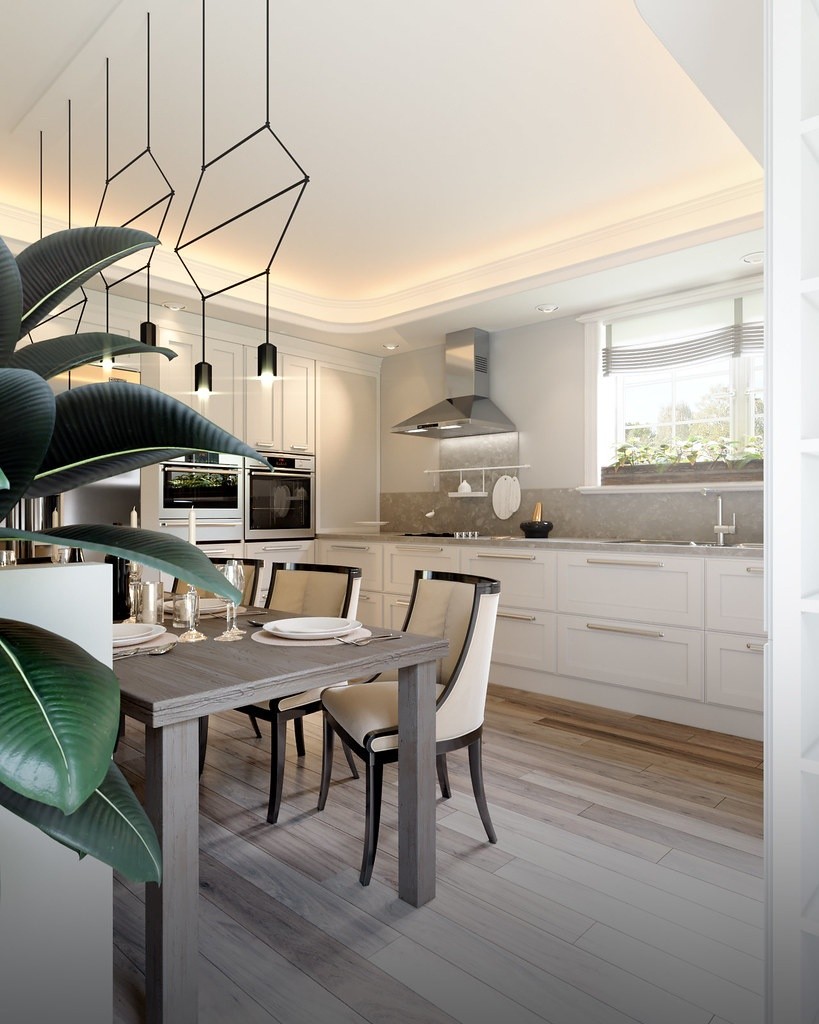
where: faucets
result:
[700,487,736,548]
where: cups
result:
[172,595,201,628]
[138,582,165,625]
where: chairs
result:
[119,557,265,779]
[319,569,502,887]
[198,562,363,825]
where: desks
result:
[111,603,453,1024]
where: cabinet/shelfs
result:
[140,542,243,592]
[246,541,313,607]
[381,545,459,680]
[140,325,243,454]
[558,549,703,734]
[313,539,382,628]
[704,557,763,742]
[0,561,114,1024]
[459,546,559,697]
[243,345,313,455]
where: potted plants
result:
[0,224,242,1024]
[601,435,765,484]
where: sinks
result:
[600,540,730,549]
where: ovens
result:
[139,442,245,543]
[245,450,316,538]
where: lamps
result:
[27,100,86,390]
[90,14,173,368]
[175,0,310,398]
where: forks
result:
[333,634,402,646]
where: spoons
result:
[113,642,178,661]
[248,619,265,626]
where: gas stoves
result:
[395,530,492,541]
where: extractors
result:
[389,327,516,439]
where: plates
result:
[262,617,362,639]
[354,521,390,526]
[163,598,231,614]
[113,623,167,647]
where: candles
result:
[130,505,138,580]
[132,581,165,626]
[189,505,197,545]
[51,507,60,563]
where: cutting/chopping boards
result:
[492,475,521,520]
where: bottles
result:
[57,548,69,564]
[104,522,132,621]
[458,479,471,492]
[67,547,84,563]
[0,550,17,567]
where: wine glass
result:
[214,559,248,643]
